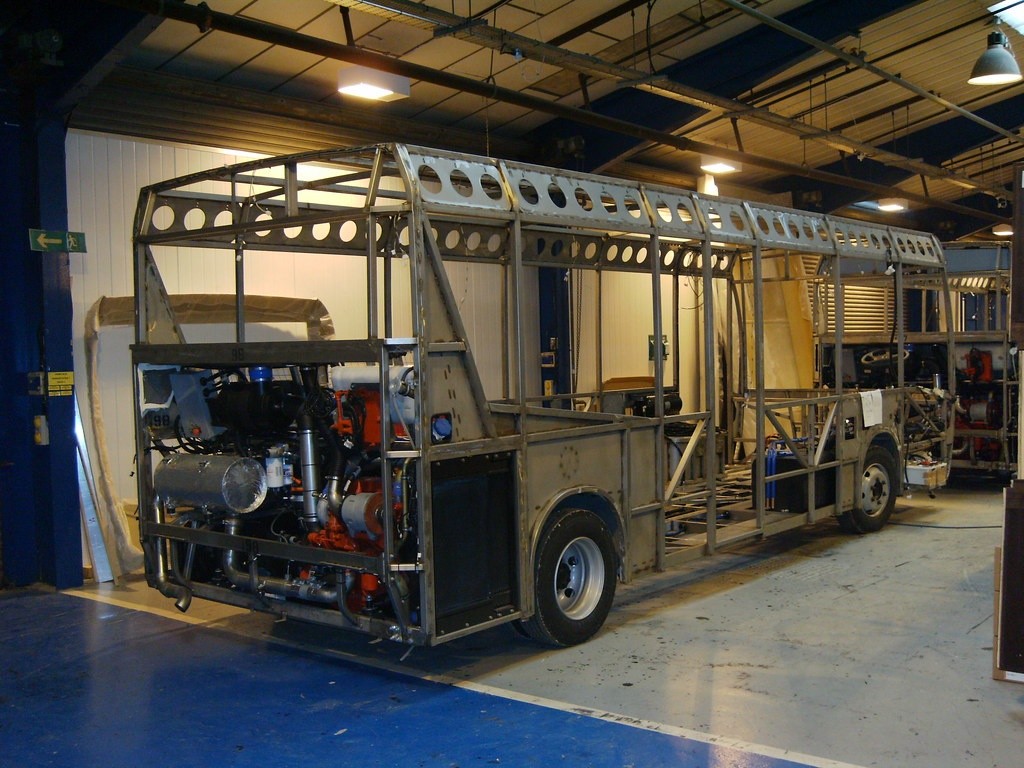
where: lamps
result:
[968,17,1022,85]
[700,154,743,174]
[992,224,1014,236]
[878,198,909,211]
[339,64,410,102]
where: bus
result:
[128,138,960,650]
